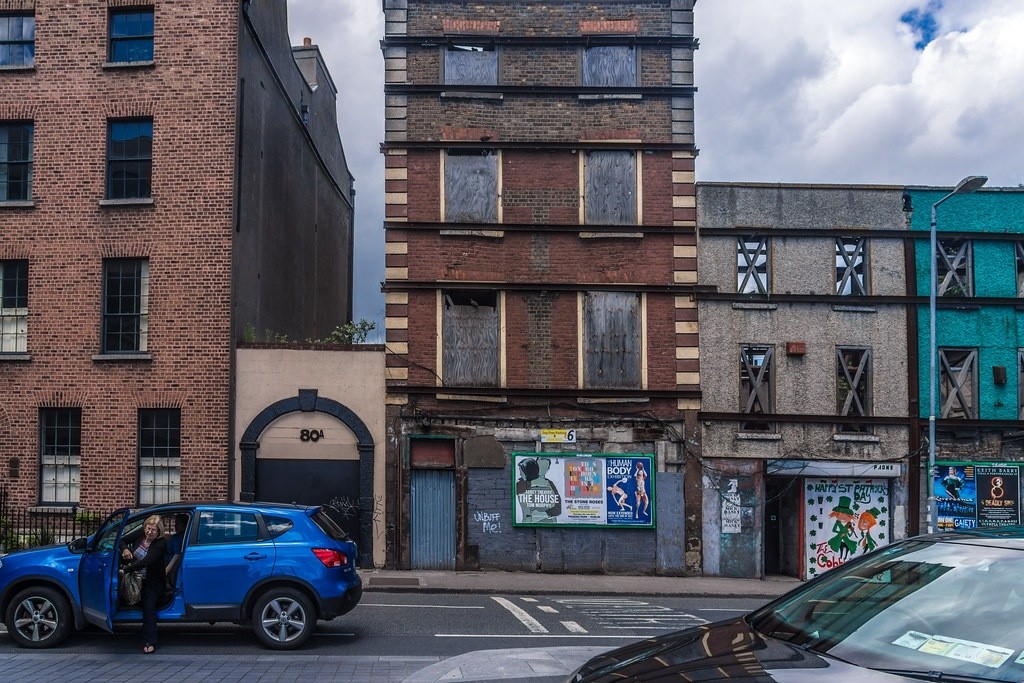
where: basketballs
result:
[636,461,643,469]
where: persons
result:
[942,466,965,498]
[118,514,189,653]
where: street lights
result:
[924,174,989,578]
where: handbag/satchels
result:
[119,560,143,605]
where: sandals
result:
[143,641,157,653]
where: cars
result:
[563,523,1024,683]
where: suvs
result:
[0,496,366,651]
